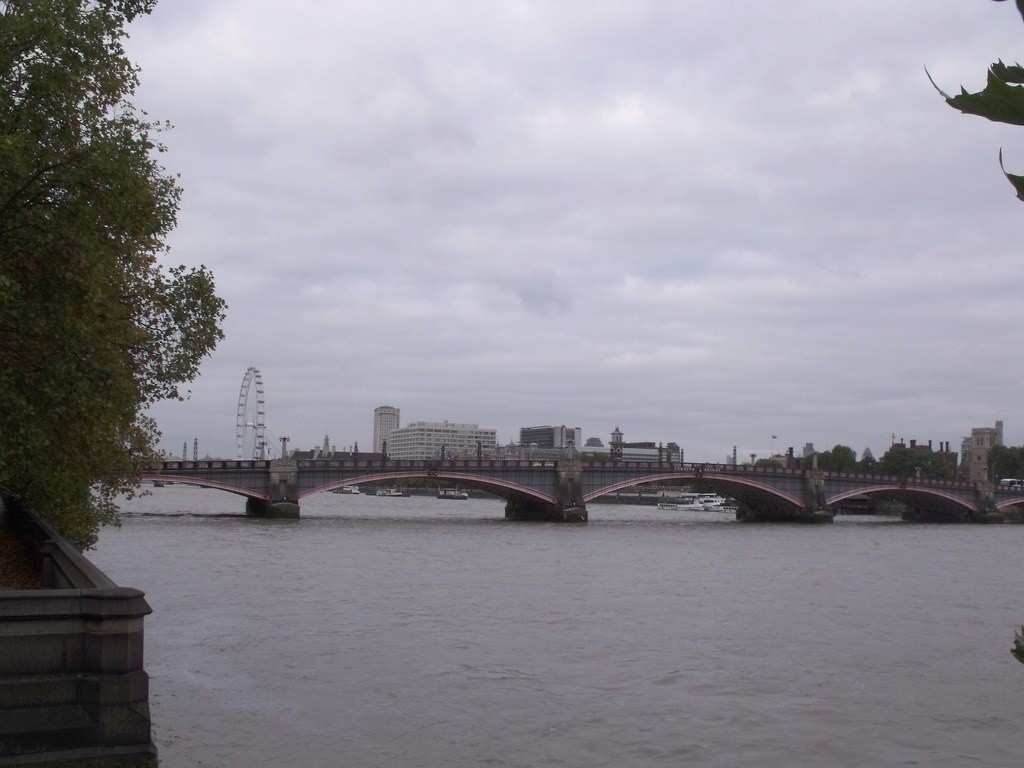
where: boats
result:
[376,487,403,496]
[677,492,726,513]
[342,484,360,494]
[437,484,468,500]
